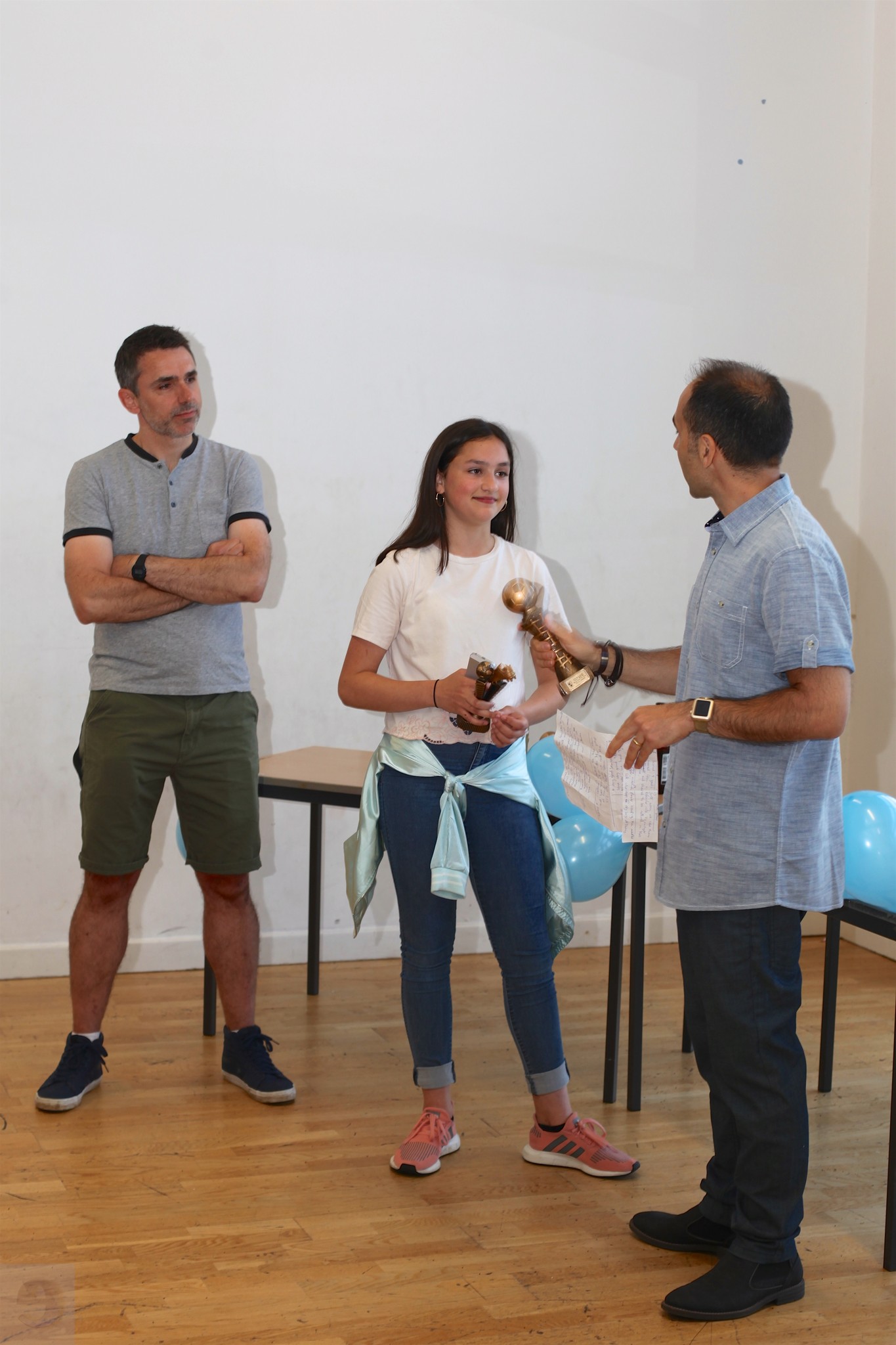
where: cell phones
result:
[486,681,509,702]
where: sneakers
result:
[34,1031,109,1110]
[390,1100,460,1174]
[662,1252,805,1320]
[221,1025,296,1103]
[629,1202,735,1259]
[522,1111,641,1176]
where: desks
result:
[203,746,633,1089]
[627,792,896,1201]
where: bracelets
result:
[434,678,440,708]
[600,640,622,685]
[580,639,608,704]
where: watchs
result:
[690,695,717,734]
[132,553,147,583]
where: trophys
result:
[501,579,595,695]
[454,661,515,735]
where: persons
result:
[32,321,297,1111]
[339,418,646,1188]
[534,355,855,1321]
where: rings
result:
[632,738,642,746]
